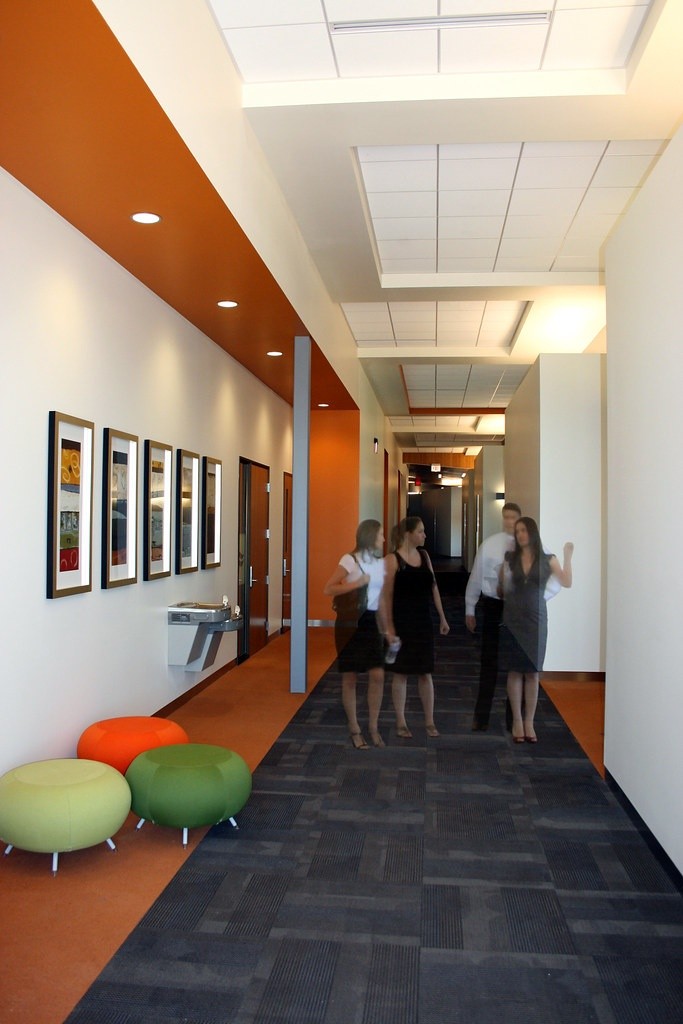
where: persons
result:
[464,501,573,744]
[323,514,450,750]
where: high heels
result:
[348,729,370,750]
[367,731,385,748]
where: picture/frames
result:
[175,449,200,576]
[46,411,94,600]
[101,428,138,590]
[143,439,173,582]
[201,457,222,570]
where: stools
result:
[0,759,131,877]
[126,744,252,849]
[77,717,188,777]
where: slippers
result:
[425,725,439,738]
[397,726,412,739]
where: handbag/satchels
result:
[332,553,368,622]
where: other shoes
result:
[525,735,538,744]
[512,733,525,743]
[473,715,490,731]
[506,722,513,732]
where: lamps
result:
[496,493,504,499]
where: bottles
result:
[385,636,401,664]
